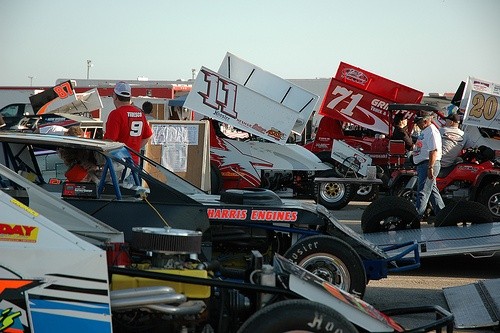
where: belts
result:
[417,160,429,166]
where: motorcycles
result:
[360,145,500,233]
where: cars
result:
[0,51,500,333]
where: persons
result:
[392,109,468,217]
[33,81,180,186]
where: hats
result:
[443,113,460,124]
[412,115,429,125]
[393,112,406,125]
[114,80,131,97]
